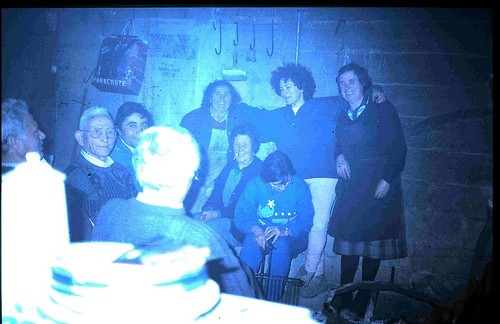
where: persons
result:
[234,151,316,301]
[0,79,263,299]
[234,63,386,298]
[323,62,407,324]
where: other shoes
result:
[351,289,374,319]
[322,291,354,315]
[302,273,329,298]
[287,266,316,298]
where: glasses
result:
[273,178,295,188]
[79,127,117,139]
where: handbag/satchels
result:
[91,34,149,96]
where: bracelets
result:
[337,161,344,167]
